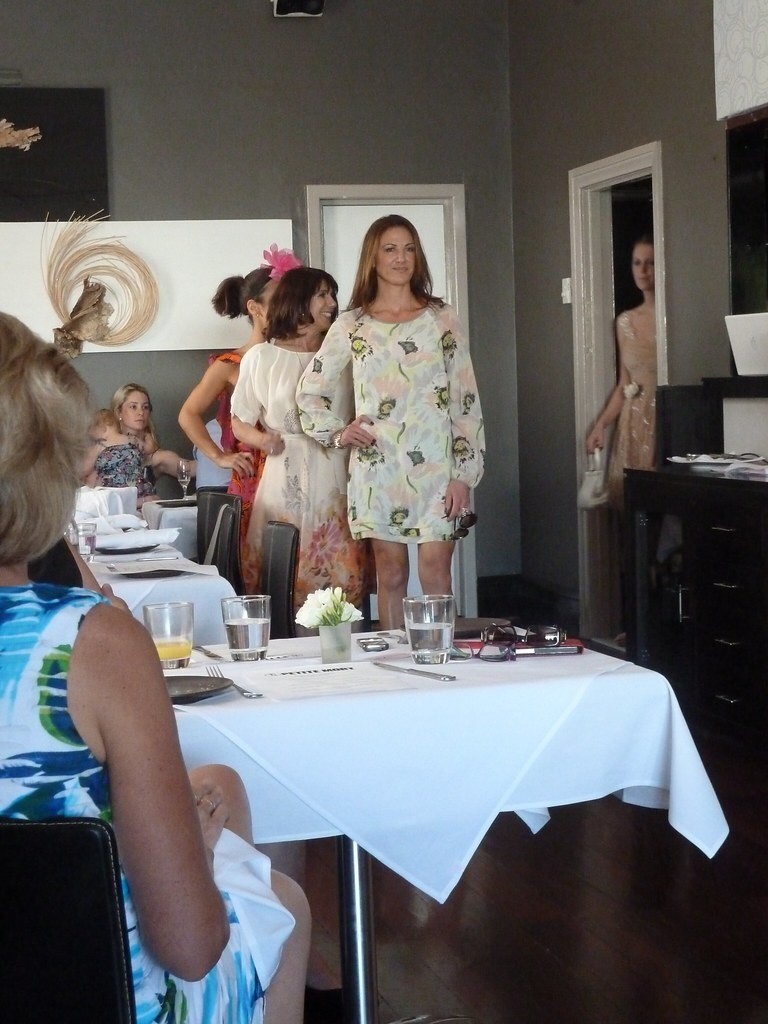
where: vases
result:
[318,622,352,664]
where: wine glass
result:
[176,460,192,500]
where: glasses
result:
[449,640,517,662]
[441,497,478,541]
[481,621,567,648]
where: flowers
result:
[295,583,364,628]
[261,243,303,279]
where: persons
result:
[74,381,231,510]
[587,240,658,645]
[292,211,487,633]
[230,265,378,618]
[0,307,311,1024]
[176,244,304,556]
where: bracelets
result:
[334,426,345,449]
[142,497,145,502]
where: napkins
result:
[92,512,149,529]
[96,524,182,549]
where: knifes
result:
[192,645,223,659]
[372,662,456,682]
[101,557,177,563]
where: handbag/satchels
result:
[578,449,610,508]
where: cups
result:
[402,594,456,665]
[68,523,97,563]
[221,595,271,661]
[143,602,194,669]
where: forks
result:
[206,665,263,698]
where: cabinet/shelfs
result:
[620,464,768,751]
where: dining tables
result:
[164,623,732,1024]
[65,518,247,647]
[143,496,198,561]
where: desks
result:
[76,486,139,516]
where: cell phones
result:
[357,638,389,652]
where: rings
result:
[271,449,273,453]
[194,793,199,801]
[201,799,216,809]
[461,507,469,518]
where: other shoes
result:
[615,632,627,648]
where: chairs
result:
[211,503,238,572]
[0,815,138,1024]
[196,486,253,585]
[253,520,299,639]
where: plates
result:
[164,676,233,703]
[156,500,197,508]
[665,455,764,469]
